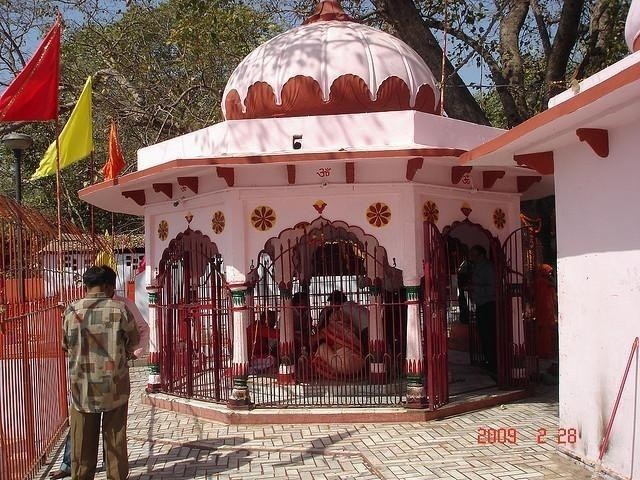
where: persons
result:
[49,265,150,479]
[292,290,370,382]
[459,245,497,375]
[536,263,558,376]
[61,266,141,479]
[247,310,280,373]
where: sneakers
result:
[53,470,71,479]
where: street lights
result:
[3,133,37,463]
